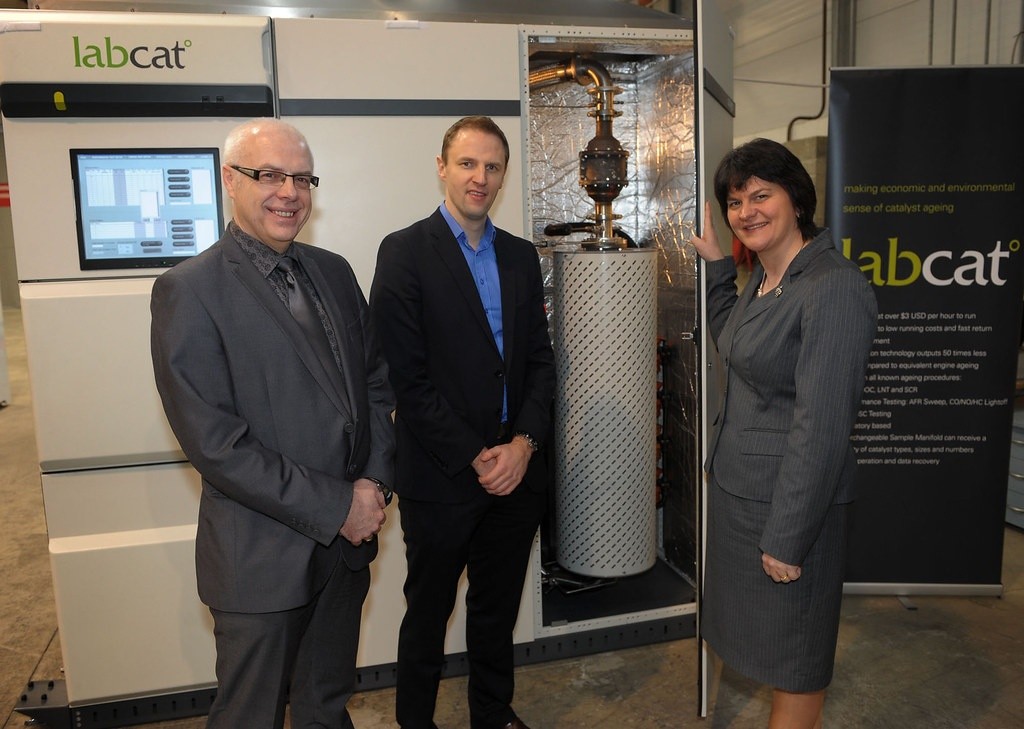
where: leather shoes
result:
[494,715,531,729]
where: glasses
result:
[232,165,319,191]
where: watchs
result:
[516,432,537,449]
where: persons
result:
[688,138,878,729]
[368,117,558,729]
[150,118,395,729]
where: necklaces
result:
[757,242,805,297]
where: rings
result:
[366,539,371,541]
[781,576,787,580]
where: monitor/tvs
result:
[70,147,225,271]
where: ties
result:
[277,256,352,418]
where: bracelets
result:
[370,477,391,499]
